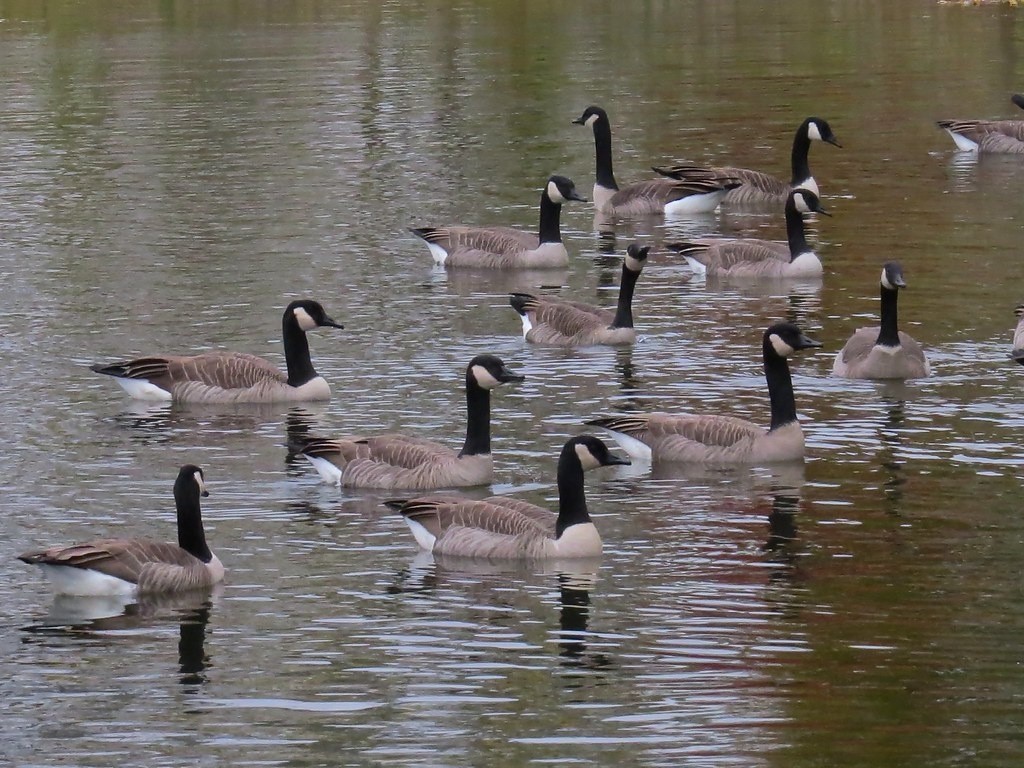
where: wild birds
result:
[382,435,633,565]
[936,93,1024,155]
[571,104,743,218]
[14,463,225,596]
[832,260,932,379]
[579,319,824,462]
[508,243,651,350]
[282,353,527,491]
[91,298,346,405]
[664,187,833,281]
[408,175,589,270]
[1005,303,1024,369]
[651,115,844,209]
